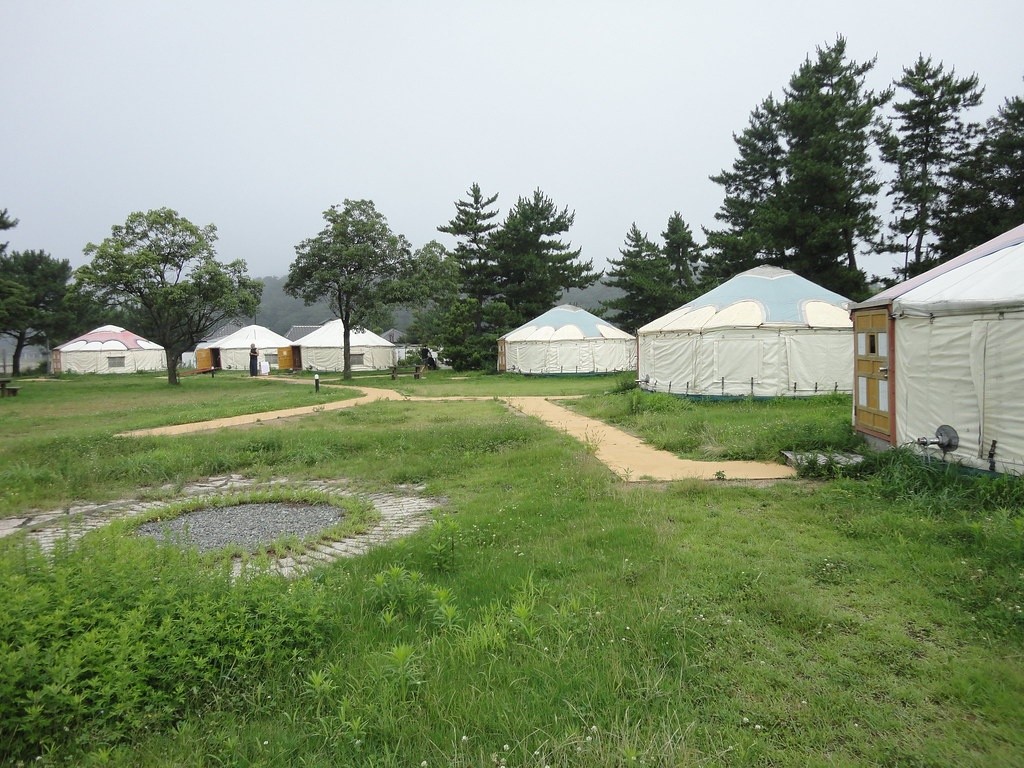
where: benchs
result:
[0,387,21,397]
[387,371,423,379]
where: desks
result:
[0,379,12,397]
[388,365,424,379]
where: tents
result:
[50,325,167,374]
[496,304,637,376]
[637,264,857,401]
[848,223,1024,477]
[196,325,293,370]
[278,319,396,373]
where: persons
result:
[249,343,259,377]
[420,344,431,373]
